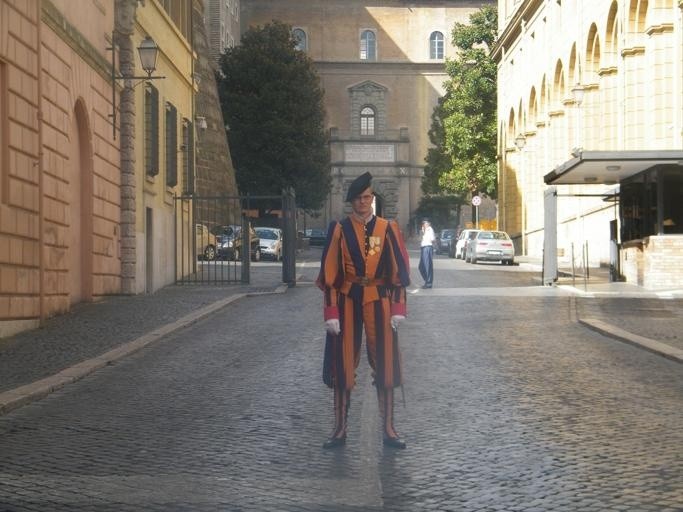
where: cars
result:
[210,225,261,262]
[300,228,327,245]
[435,229,514,265]
[252,227,284,260]
[195,223,217,260]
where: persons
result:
[418,217,436,289]
[316,172,411,450]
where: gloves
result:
[390,314,405,332]
[324,319,341,336]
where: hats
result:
[345,169,372,202]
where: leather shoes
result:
[383,437,406,450]
[323,437,346,449]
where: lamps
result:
[511,132,526,152]
[194,115,207,131]
[101,29,168,143]
[583,177,597,184]
[606,166,620,172]
